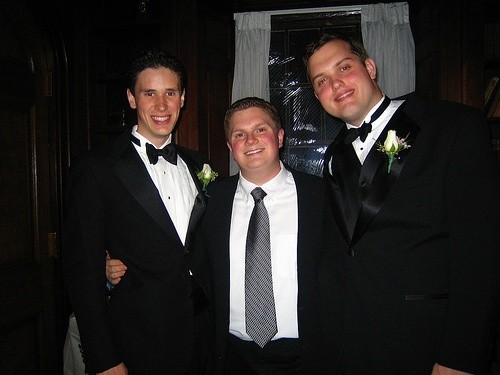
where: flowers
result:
[195,162,220,192]
[372,130,411,173]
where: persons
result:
[105,97,347,375]
[303,30,500,375]
[74,44,221,375]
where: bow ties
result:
[345,94,392,144]
[127,133,177,165]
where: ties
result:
[245,187,278,349]
